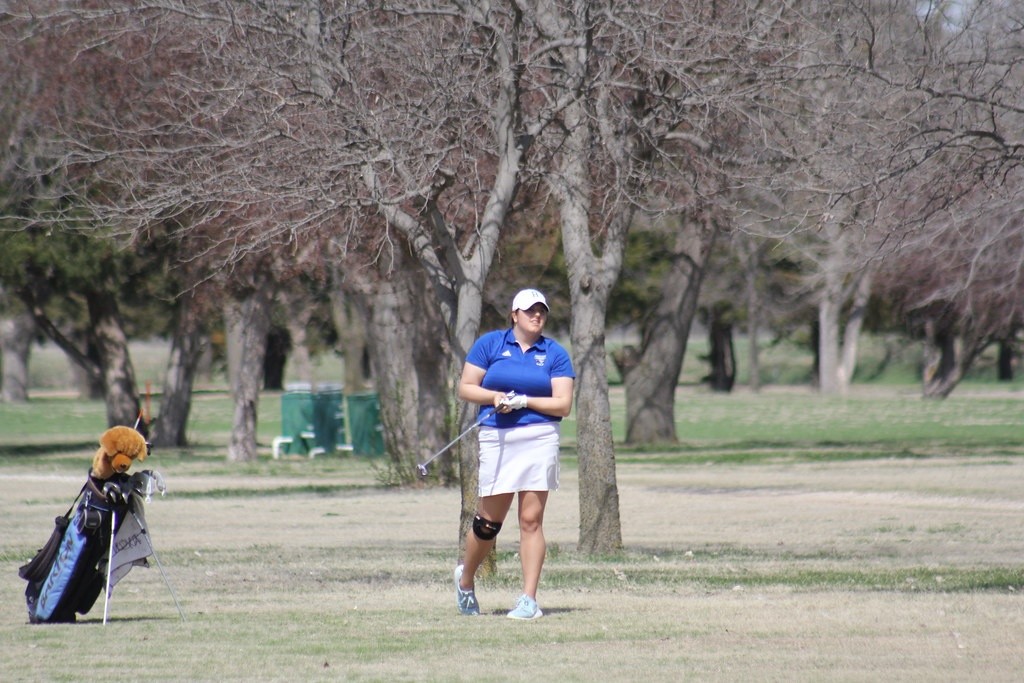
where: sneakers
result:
[455,564,480,616]
[506,594,542,620]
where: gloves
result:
[500,394,527,410]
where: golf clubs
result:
[119,484,188,624]
[414,390,515,477]
[100,480,121,627]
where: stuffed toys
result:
[90,424,148,480]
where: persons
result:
[452,288,576,621]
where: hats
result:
[512,289,550,312]
